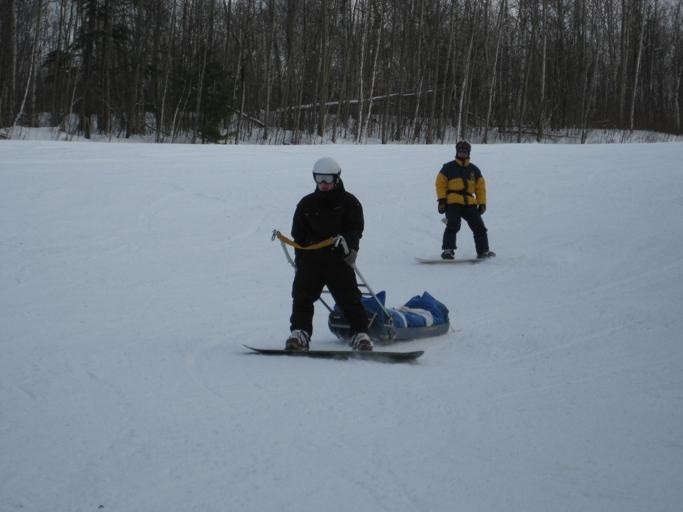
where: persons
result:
[435,140,496,260]
[285,156,375,351]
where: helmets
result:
[312,156,341,175]
[456,141,471,150]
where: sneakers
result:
[476,251,496,258]
[285,329,310,351]
[351,332,373,351]
[441,250,455,259]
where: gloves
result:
[343,249,358,266]
[479,204,486,214]
[438,199,447,214]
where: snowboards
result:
[242,345,425,362]
[414,257,487,264]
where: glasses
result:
[313,174,338,184]
